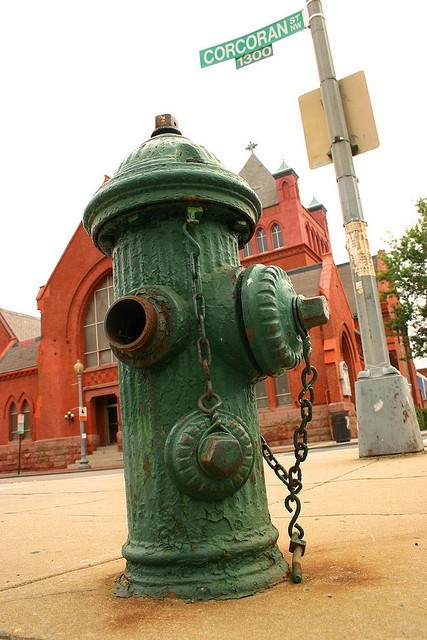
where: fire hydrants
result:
[82,114,330,601]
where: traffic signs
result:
[199,9,305,70]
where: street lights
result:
[74,359,91,469]
